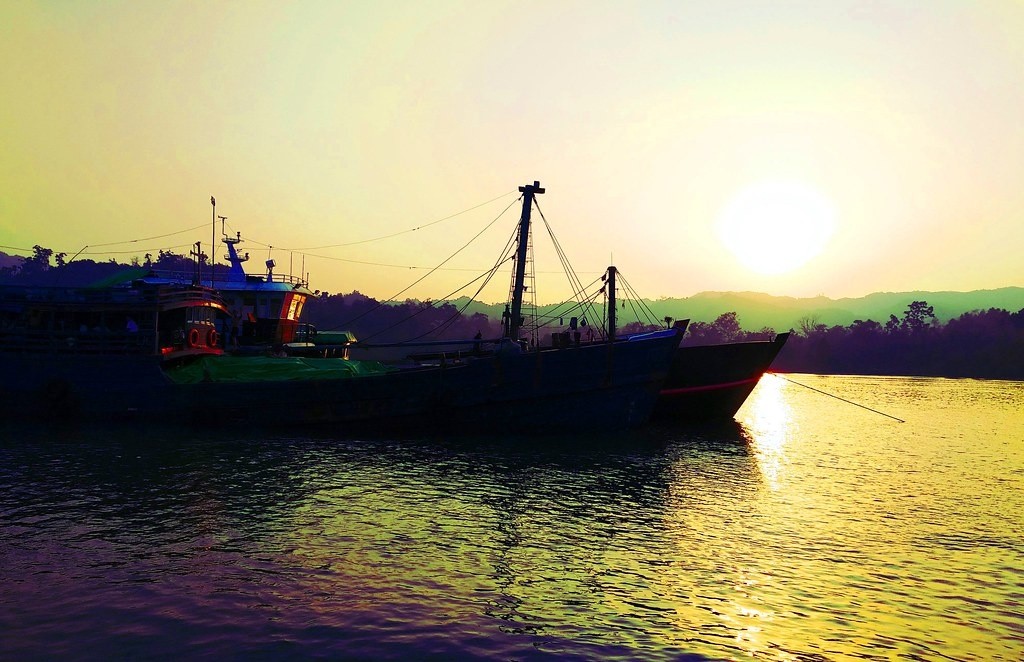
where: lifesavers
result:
[207,328,218,347]
[188,330,199,346]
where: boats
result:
[71,180,792,429]
